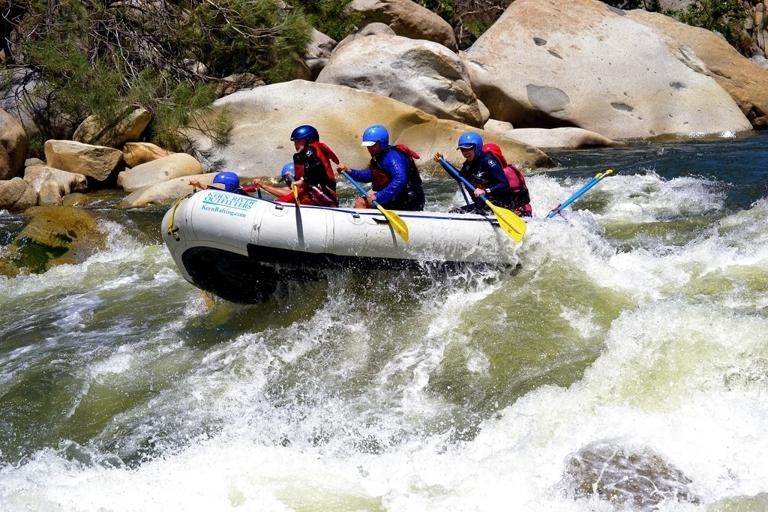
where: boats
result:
[160,187,540,307]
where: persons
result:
[252,124,339,209]
[336,126,426,212]
[433,131,533,217]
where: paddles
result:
[439,158,526,242]
[342,169,409,241]
[289,175,301,209]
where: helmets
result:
[278,163,295,182]
[361,125,389,151]
[207,172,238,192]
[456,132,505,165]
[291,126,319,141]
